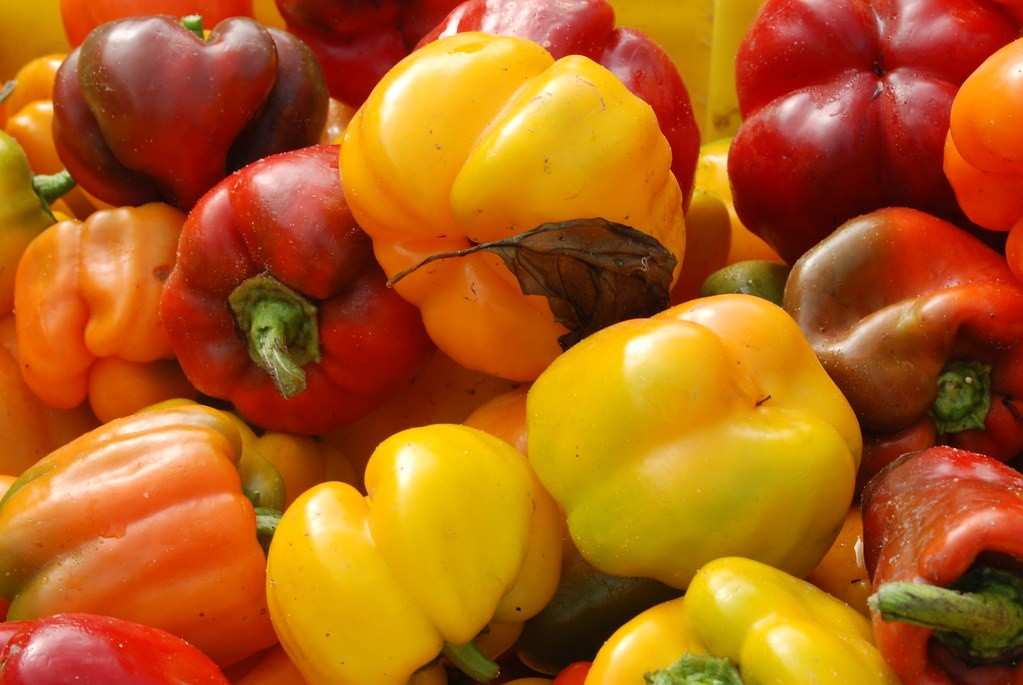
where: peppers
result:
[0,0,1023,685]
